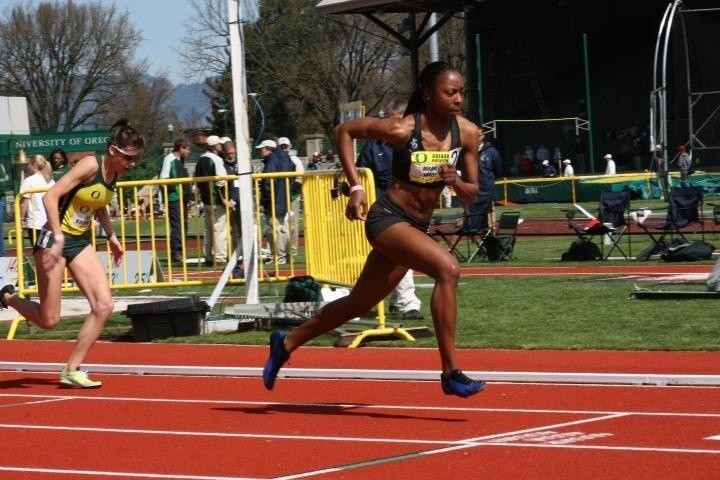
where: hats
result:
[563,159,571,164]
[542,159,549,165]
[604,154,613,159]
[254,137,291,148]
[206,135,232,146]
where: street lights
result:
[168,123,175,143]
[218,108,229,137]
[248,90,265,145]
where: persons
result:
[0,118,148,388]
[156,134,305,265]
[308,151,323,170]
[326,149,336,163]
[0,164,11,258]
[18,155,55,247]
[263,60,487,398]
[456,126,501,228]
[355,96,426,320]
[511,124,654,178]
[676,145,692,188]
[48,149,73,182]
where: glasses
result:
[113,145,141,161]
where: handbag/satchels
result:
[283,276,323,302]
[562,237,602,262]
[660,238,716,262]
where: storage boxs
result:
[119,294,212,343]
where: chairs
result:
[428,199,494,263]
[627,185,706,261]
[567,189,632,261]
[480,209,522,262]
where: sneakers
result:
[441,369,487,398]
[58,371,102,389]
[389,304,424,320]
[264,329,291,389]
[0,283,15,309]
[264,256,296,265]
